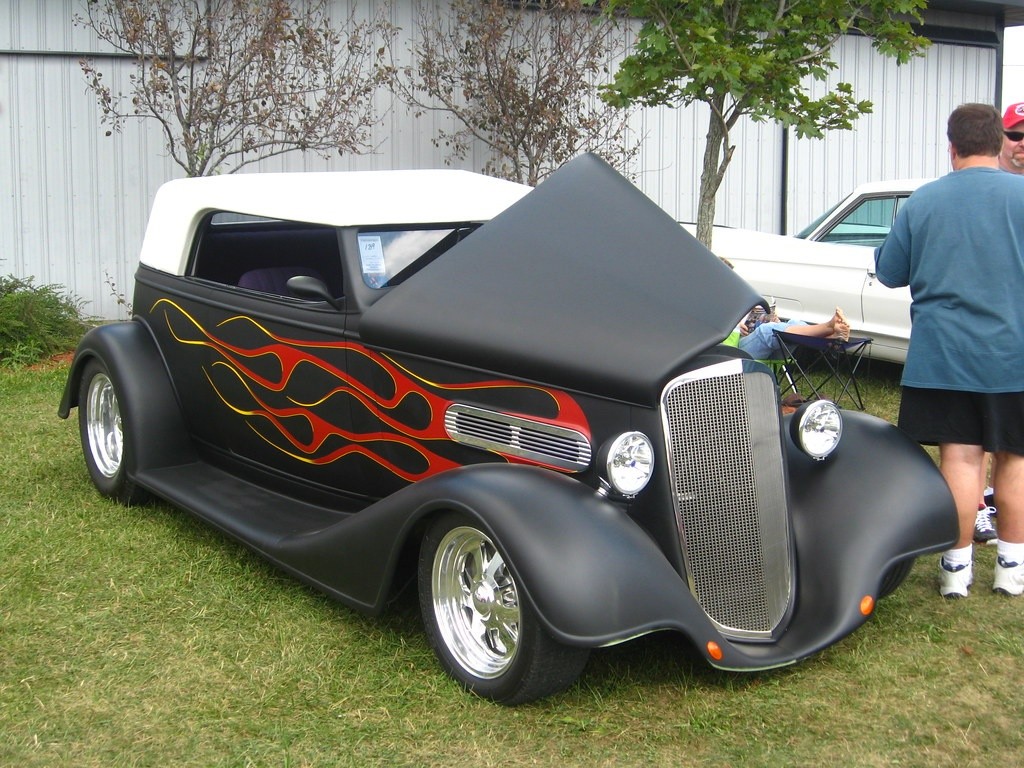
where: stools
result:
[772,322,874,412]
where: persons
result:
[873,103,1024,598]
[717,257,847,358]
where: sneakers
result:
[991,556,1023,597]
[984,493,997,517]
[975,505,997,545]
[937,556,973,598]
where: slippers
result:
[815,394,828,401]
[781,392,804,407]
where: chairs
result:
[237,264,330,301]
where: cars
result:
[676,178,940,372]
[57,154,959,706]
